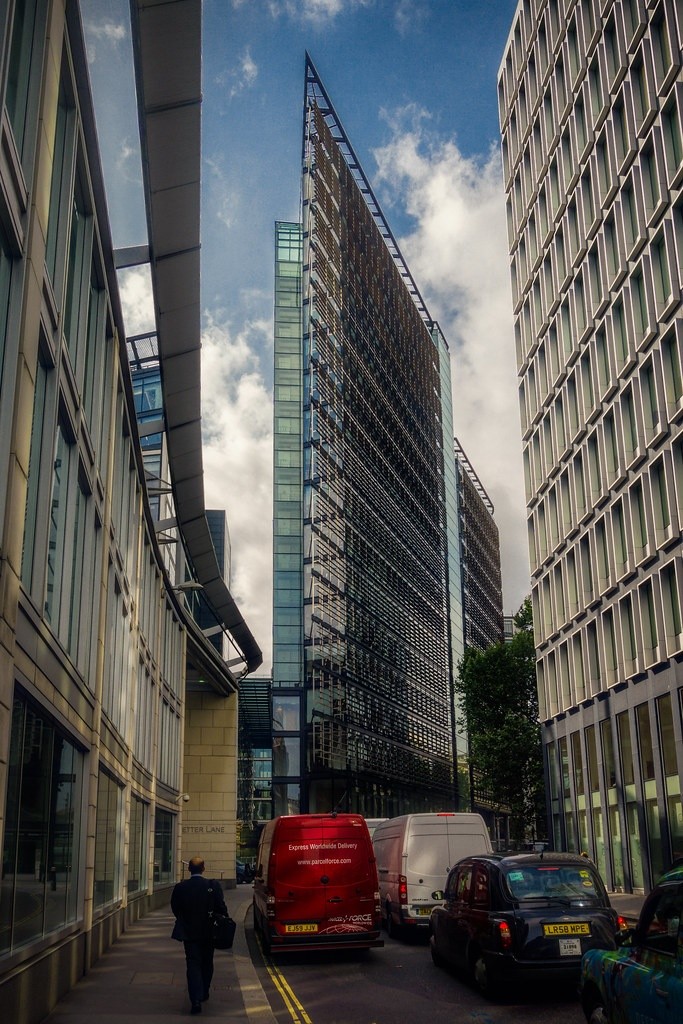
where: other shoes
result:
[202,992,209,1001]
[191,1006,201,1014]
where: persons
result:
[171,856,229,1015]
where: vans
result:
[367,810,494,936]
[244,811,386,965]
[362,817,392,843]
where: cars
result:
[425,850,630,1006]
[236,859,258,885]
[574,863,683,1024]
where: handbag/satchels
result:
[212,914,236,949]
